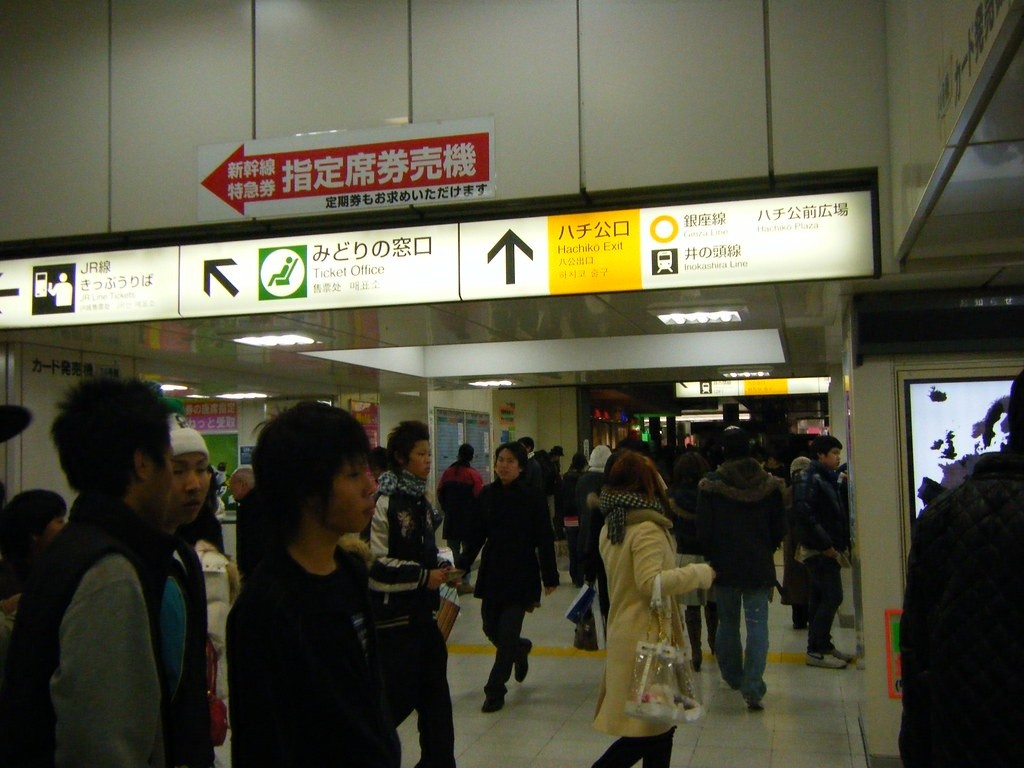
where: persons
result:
[0,404,67,673]
[696,427,789,710]
[589,452,717,767]
[517,436,612,651]
[897,370,1024,768]
[437,444,482,595]
[0,378,218,768]
[444,442,561,713]
[616,434,851,668]
[155,397,277,768]
[225,400,400,767]
[364,421,462,767]
[358,446,389,546]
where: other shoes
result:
[514,639,532,682]
[457,584,475,594]
[482,696,504,712]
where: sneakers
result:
[806,653,847,668]
[829,648,852,662]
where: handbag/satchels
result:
[565,584,596,623]
[573,605,599,652]
[205,638,228,746]
[433,582,461,640]
[623,573,703,722]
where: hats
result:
[550,446,564,456]
[589,445,612,467]
[170,396,209,459]
